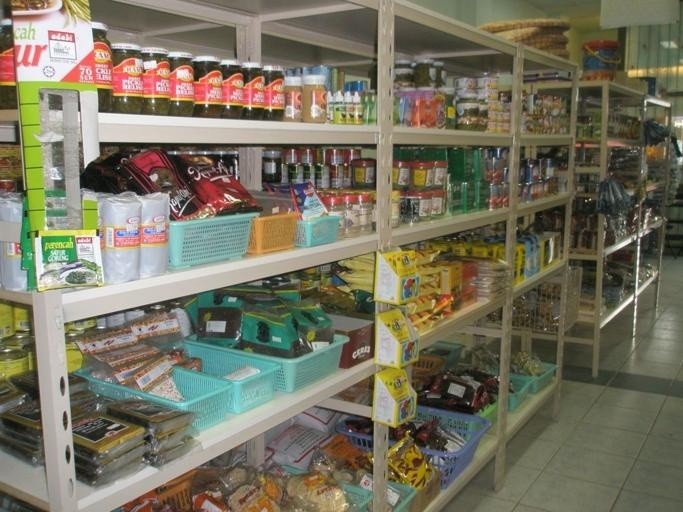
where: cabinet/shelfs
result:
[1,0,385,511]
[390,2,518,511]
[502,41,681,492]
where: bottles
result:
[319,192,372,239]
[327,90,363,125]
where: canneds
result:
[92,21,327,123]
[395,59,569,135]
[262,144,555,239]
[0,17,18,110]
[207,151,241,180]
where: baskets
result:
[282,464,372,511]
[491,266,583,333]
[247,211,300,254]
[478,403,497,424]
[518,363,559,394]
[168,211,258,267]
[486,371,532,409]
[387,481,417,511]
[336,404,492,490]
[295,215,340,248]
[274,338,560,512]
[152,479,193,512]
[435,342,465,366]
[413,354,445,385]
[73,316,350,430]
